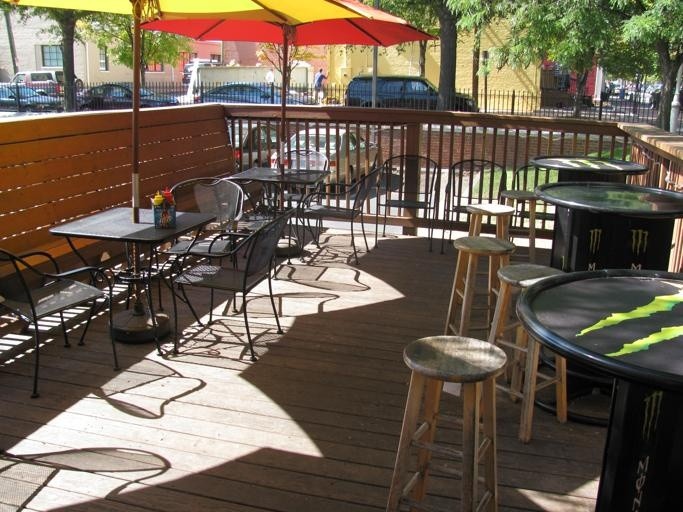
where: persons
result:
[265,68,276,87]
[312,67,329,104]
[603,78,636,102]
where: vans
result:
[10,71,83,96]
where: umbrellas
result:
[0,0,372,223]
[139,0,439,176]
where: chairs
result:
[507,165,558,260]
[375,154,440,252]
[2,246,123,399]
[169,203,296,362]
[440,159,507,255]
[162,177,245,315]
[213,151,381,272]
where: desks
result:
[535,181,683,429]
[48,205,219,357]
[518,268,683,512]
[530,155,649,183]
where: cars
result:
[194,83,317,105]
[76,83,180,111]
[227,123,286,172]
[271,128,380,192]
[0,83,62,113]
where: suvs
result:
[345,76,477,111]
[181,59,223,88]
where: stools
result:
[496,190,538,270]
[386,335,509,512]
[457,203,516,305]
[487,263,568,443]
[444,235,516,337]
